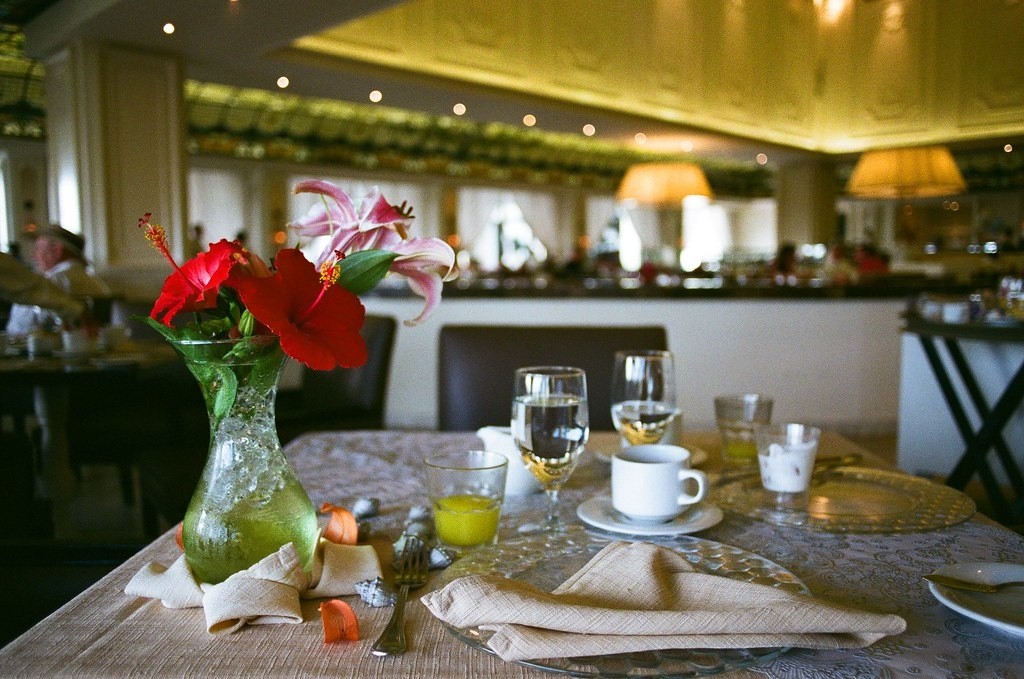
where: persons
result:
[562,247,588,276]
[6,225,113,470]
[188,221,206,257]
[855,246,889,277]
[775,242,799,276]
[0,251,100,338]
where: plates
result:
[928,561,1024,636]
[431,526,813,679]
[594,444,708,467]
[576,495,724,536]
[704,465,977,531]
[51,349,99,359]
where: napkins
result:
[123,528,384,635]
[420,540,907,662]
[473,424,546,498]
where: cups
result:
[61,328,89,351]
[754,422,821,511]
[424,450,509,558]
[714,394,773,470]
[611,444,708,524]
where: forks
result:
[370,536,428,655]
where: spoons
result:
[921,574,1024,594]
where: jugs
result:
[477,425,540,496]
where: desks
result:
[0,342,177,500]
[900,320,1024,528]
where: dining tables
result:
[0,431,1024,678]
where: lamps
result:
[843,144,967,199]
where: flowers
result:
[137,179,461,457]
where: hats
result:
[24,224,90,267]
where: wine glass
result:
[610,350,676,446]
[511,366,589,540]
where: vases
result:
[171,337,318,585]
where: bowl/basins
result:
[943,302,969,324]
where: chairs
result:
[439,325,667,432]
[273,313,398,446]
